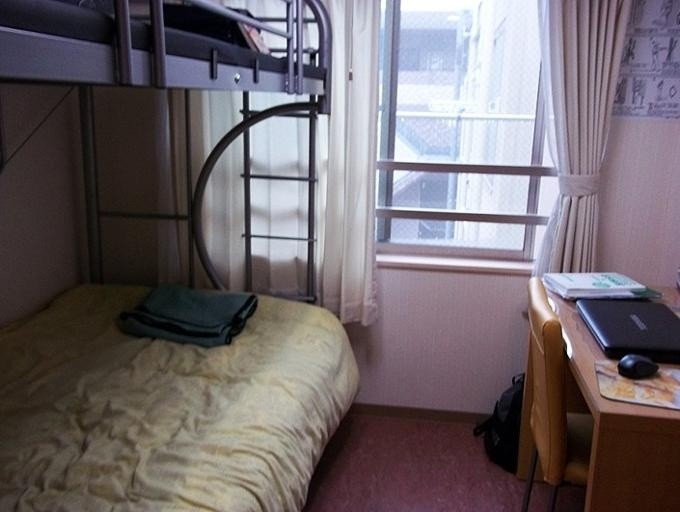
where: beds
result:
[0,0,333,510]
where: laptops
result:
[575,299,680,361]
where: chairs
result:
[523,278,596,512]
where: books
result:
[542,271,663,300]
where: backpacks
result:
[473,373,525,474]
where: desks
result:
[518,274,680,512]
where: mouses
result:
[618,353,660,379]
